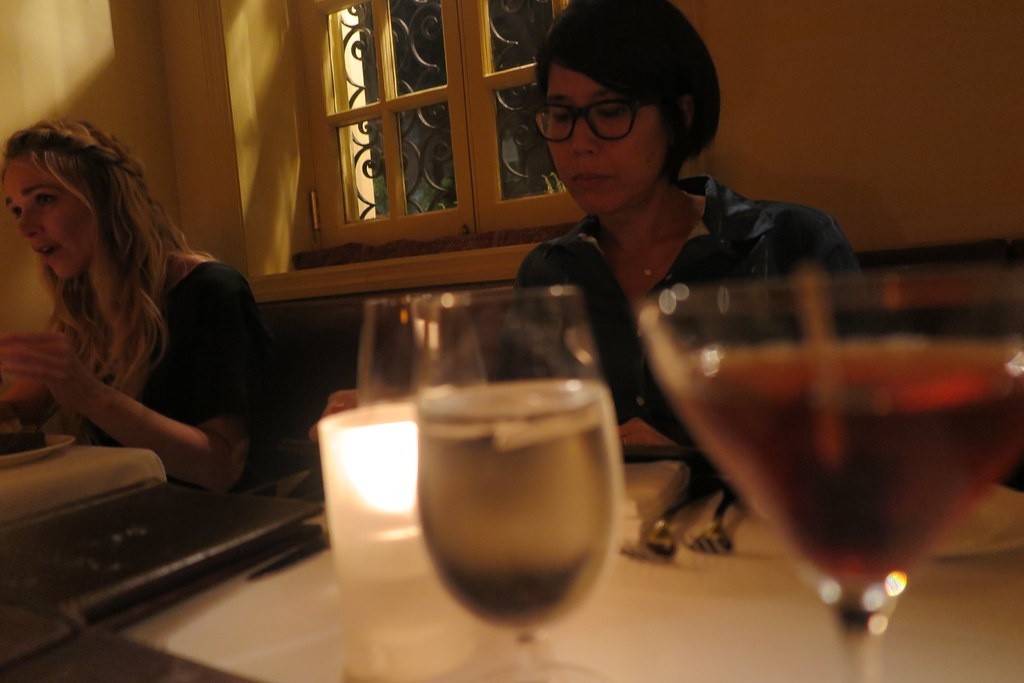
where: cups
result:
[357,296,486,410]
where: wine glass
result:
[417,286,623,682]
[633,271,1024,683]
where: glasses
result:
[534,97,661,142]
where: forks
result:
[621,478,721,564]
[681,486,737,554]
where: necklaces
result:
[604,209,701,279]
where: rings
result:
[620,435,629,449]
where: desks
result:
[108,459,1023,683]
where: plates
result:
[0,435,75,464]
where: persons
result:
[0,114,270,499]
[508,0,881,452]
